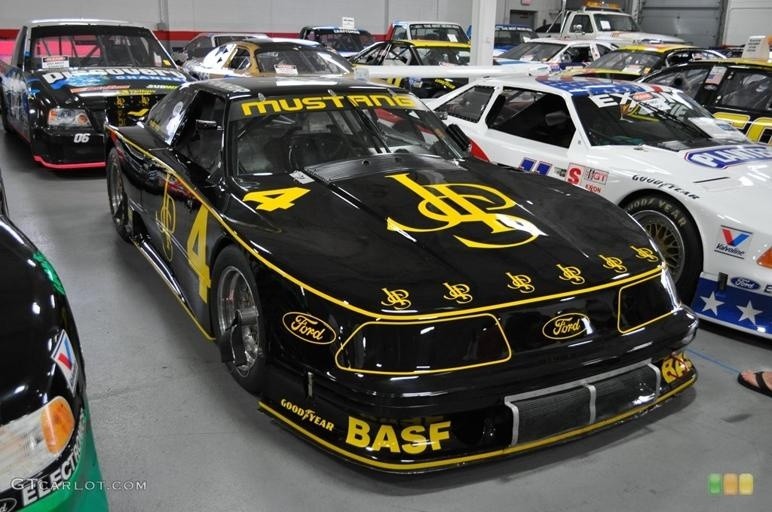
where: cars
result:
[415,78,771,343]
[0,168,109,512]
[103,76,696,475]
[635,59,772,143]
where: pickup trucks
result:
[532,1,697,52]
[1,18,188,171]
